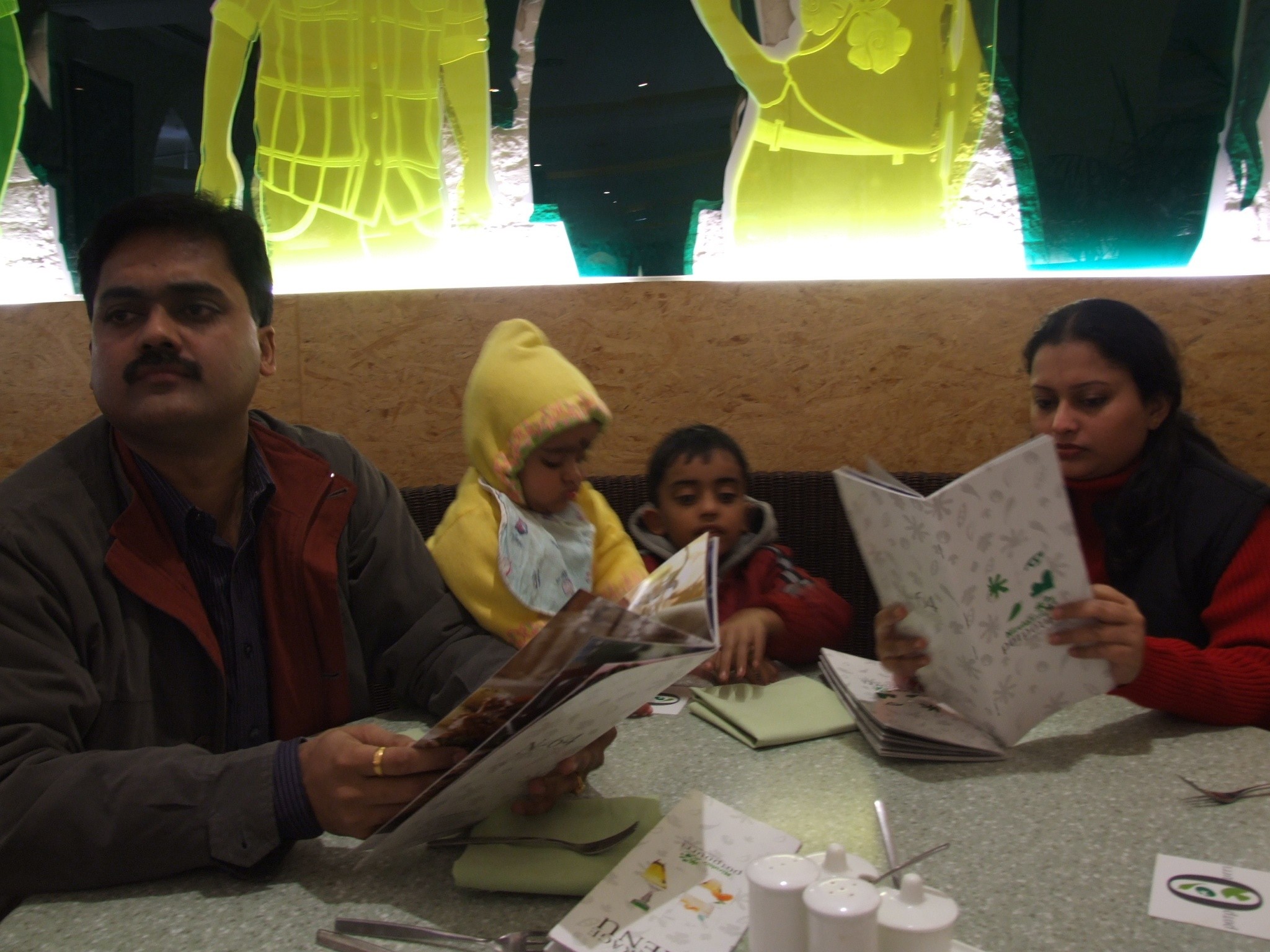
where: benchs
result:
[394,469,953,668]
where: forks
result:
[1175,773,1270,803]
[335,916,550,951]
[427,819,642,857]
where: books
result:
[817,648,1005,762]
[374,531,720,852]
[833,435,1113,749]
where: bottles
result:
[875,875,959,952]
[746,853,818,952]
[802,876,882,952]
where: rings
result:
[373,747,385,777]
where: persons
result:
[425,319,647,649]
[627,425,851,683]
[1,197,622,895]
[877,298,1270,733]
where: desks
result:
[0,669,1269,952]
